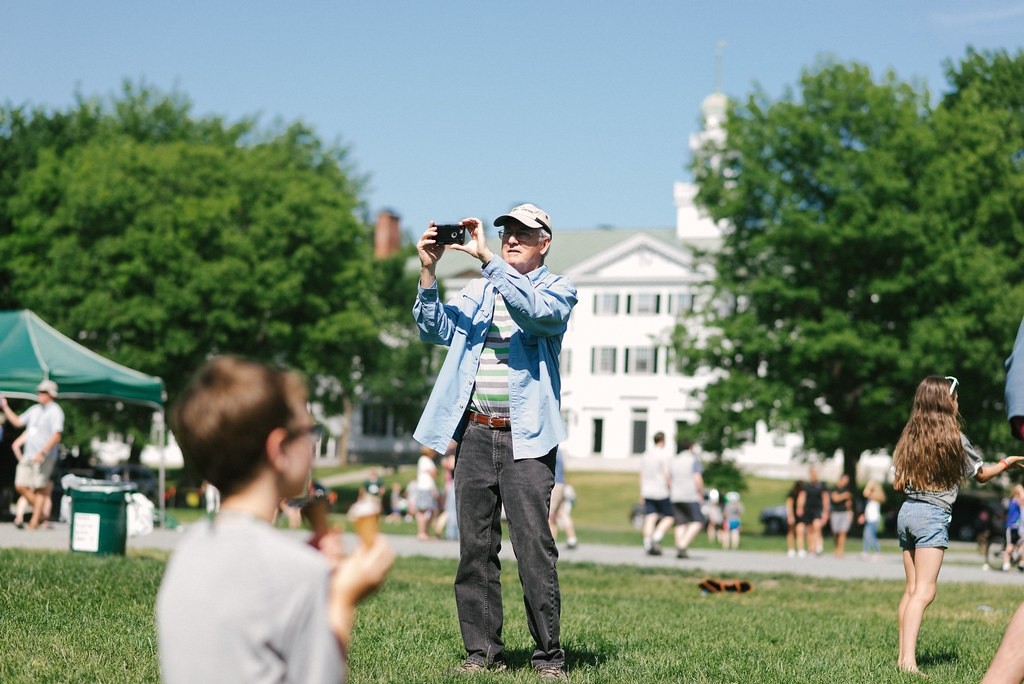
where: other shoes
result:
[538,667,566,680]
[455,662,488,674]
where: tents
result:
[0,310,166,526]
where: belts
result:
[463,410,511,428]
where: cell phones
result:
[431,224,466,245]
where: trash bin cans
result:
[63,480,138,557]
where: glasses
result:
[498,229,548,243]
[945,376,960,396]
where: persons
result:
[1,381,66,527]
[640,431,705,558]
[393,443,461,540]
[787,469,886,555]
[893,376,1024,676]
[157,355,394,683]
[359,471,386,518]
[412,204,579,677]
[549,453,576,548]
[982,321,1024,684]
[702,495,745,549]
[1002,486,1024,571]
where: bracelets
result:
[41,451,47,458]
[999,459,1011,467]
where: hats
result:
[38,379,59,398]
[493,203,553,241]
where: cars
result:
[884,491,1005,541]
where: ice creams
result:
[301,498,328,538]
[346,496,380,552]
